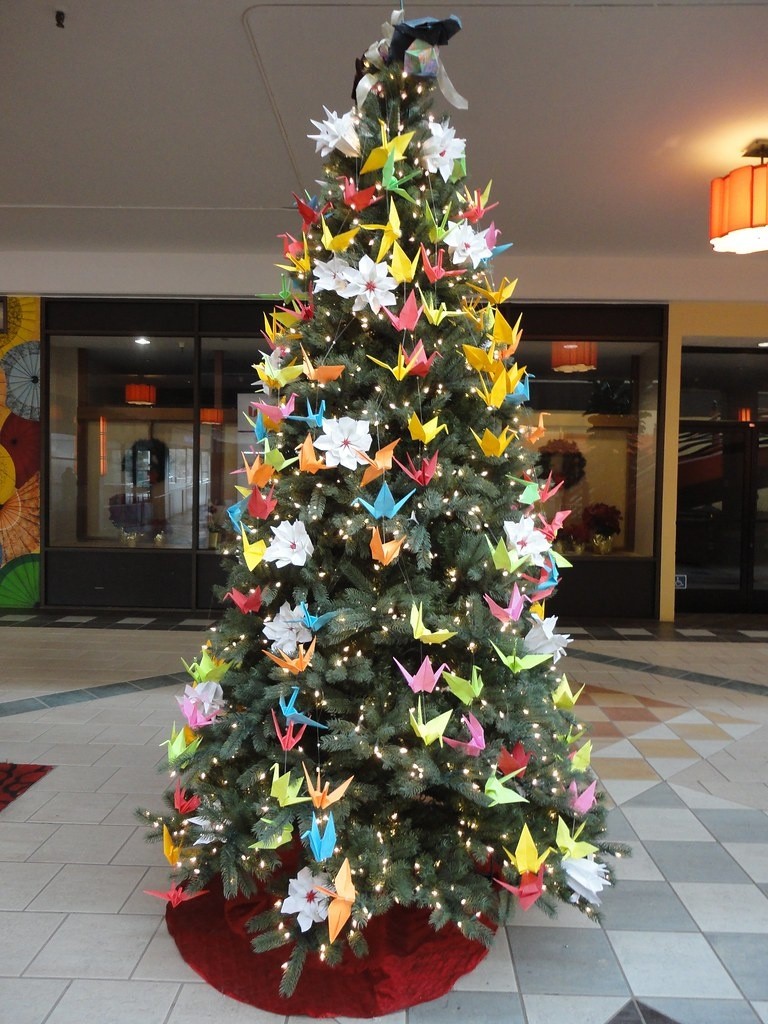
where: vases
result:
[573,541,585,554]
[122,529,143,548]
[153,531,166,546]
[209,527,218,549]
[592,533,612,554]
[551,485,570,553]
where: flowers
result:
[565,523,591,542]
[207,505,222,531]
[108,494,172,535]
[583,503,622,536]
[535,439,587,488]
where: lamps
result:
[125,337,157,406]
[708,138,768,255]
[200,408,223,425]
[551,341,598,373]
